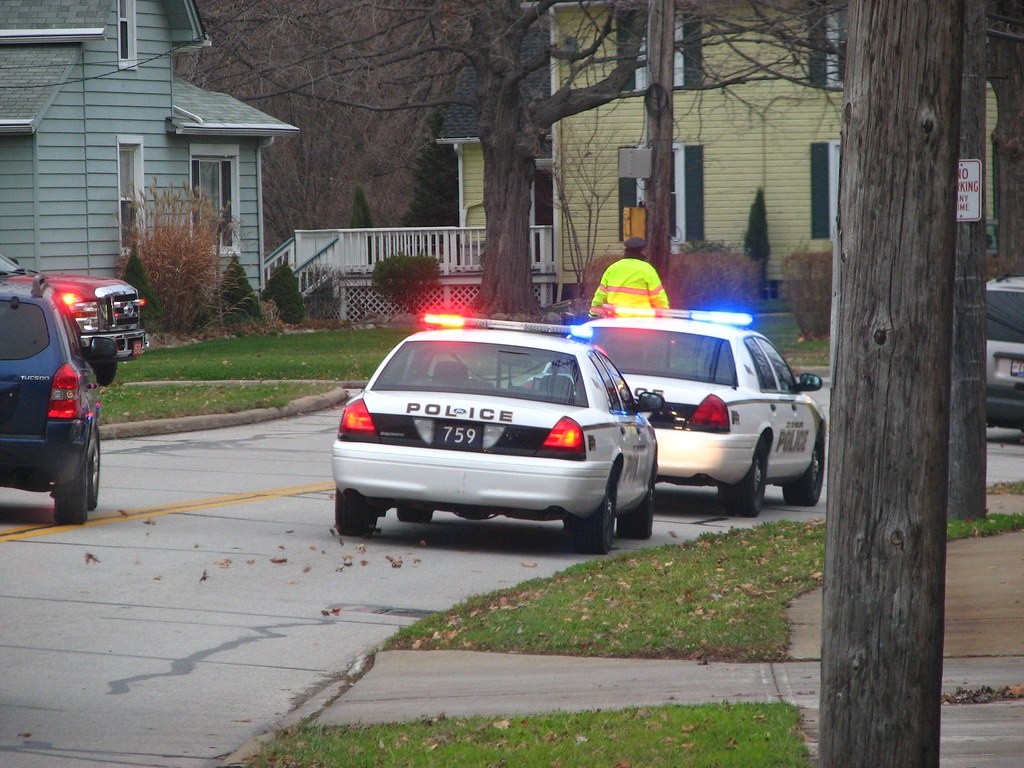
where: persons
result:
[587,237,670,320]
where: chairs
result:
[431,361,468,388]
[540,375,574,400]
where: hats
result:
[624,237,648,249]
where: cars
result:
[565,307,827,516]
[332,313,665,555]
[986,271,1024,431]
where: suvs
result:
[0,245,150,385]
[0,274,100,524]
